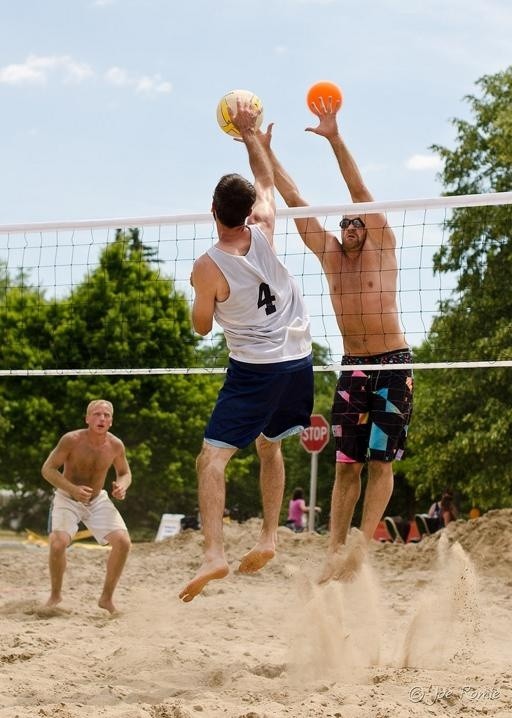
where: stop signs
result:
[298,414,331,533]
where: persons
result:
[288,488,321,529]
[429,490,484,527]
[178,96,311,601]
[231,97,413,586]
[42,399,132,620]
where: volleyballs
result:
[217,90,264,138]
[307,82,342,115]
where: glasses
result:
[337,218,364,228]
